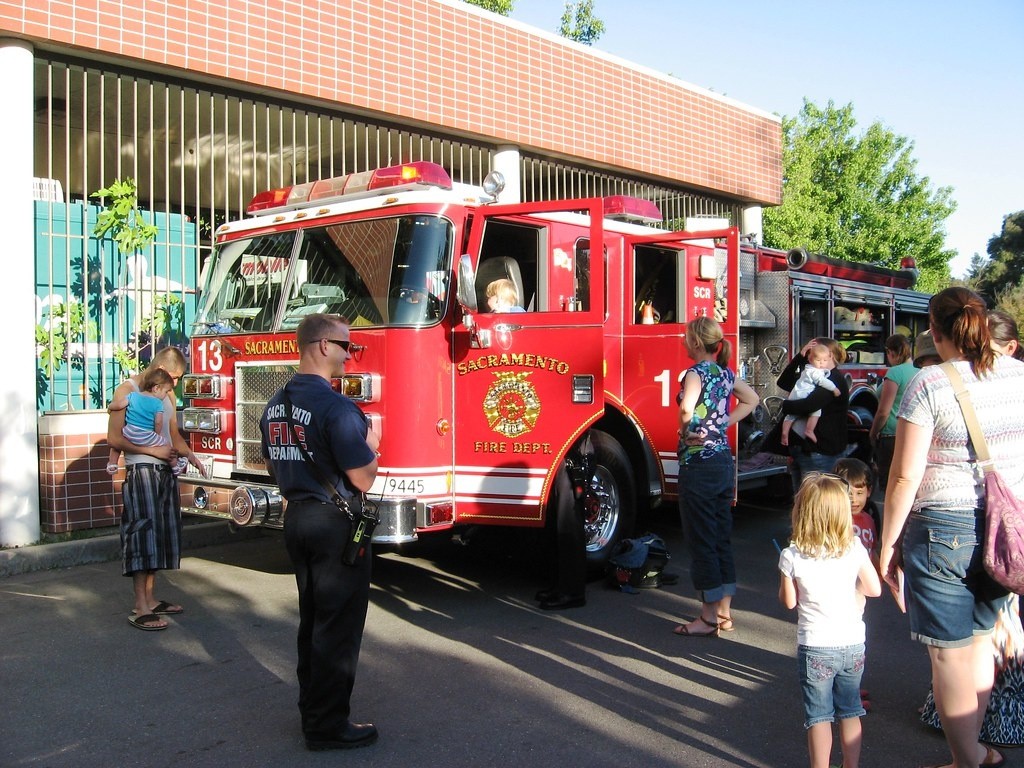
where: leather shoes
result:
[302,723,379,751]
[534,589,586,610]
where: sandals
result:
[672,615,735,637]
[106,462,118,474]
[172,457,188,476]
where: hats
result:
[910,329,938,368]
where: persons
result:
[260,315,380,750]
[106,368,188,475]
[481,280,587,610]
[670,316,759,638]
[106,347,209,632]
[776,286,1024,768]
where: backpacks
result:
[602,534,672,569]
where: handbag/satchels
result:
[984,470,1024,596]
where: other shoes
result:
[863,701,871,710]
[830,763,843,768]
[632,573,679,588]
[994,743,1023,747]
[861,690,869,697]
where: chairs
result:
[475,257,525,314]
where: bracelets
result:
[677,429,689,438]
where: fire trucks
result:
[174,161,938,581]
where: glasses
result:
[309,340,349,352]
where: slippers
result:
[978,743,1007,766]
[131,600,183,614]
[128,615,169,630]
[917,763,946,768]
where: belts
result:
[293,498,319,502]
[126,463,172,471]
[881,434,895,438]
